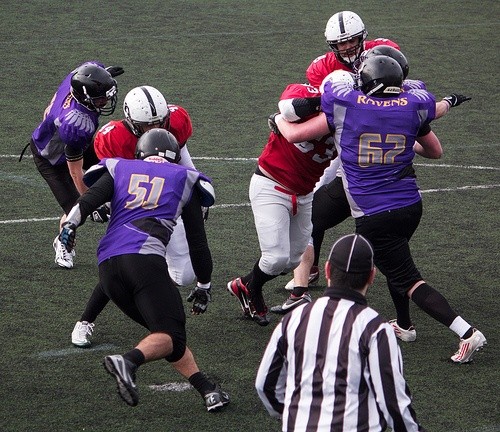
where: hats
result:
[328,234,374,273]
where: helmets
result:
[356,56,404,99]
[324,11,369,70]
[71,64,118,111]
[122,85,170,137]
[365,45,410,81]
[135,128,182,164]
[167,103,193,145]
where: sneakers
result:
[389,318,417,342]
[285,271,320,291]
[102,355,139,407]
[450,327,488,364]
[226,277,253,321]
[244,279,271,327]
[53,234,76,269]
[71,321,96,347]
[200,383,231,413]
[270,290,312,314]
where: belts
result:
[255,166,285,187]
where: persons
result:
[58,128,232,411]
[255,234,426,432]
[30,62,124,268]
[228,11,487,363]
[72,84,198,346]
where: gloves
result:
[58,222,77,253]
[267,113,282,135]
[442,93,472,113]
[90,204,110,223]
[186,285,213,315]
[105,65,125,78]
